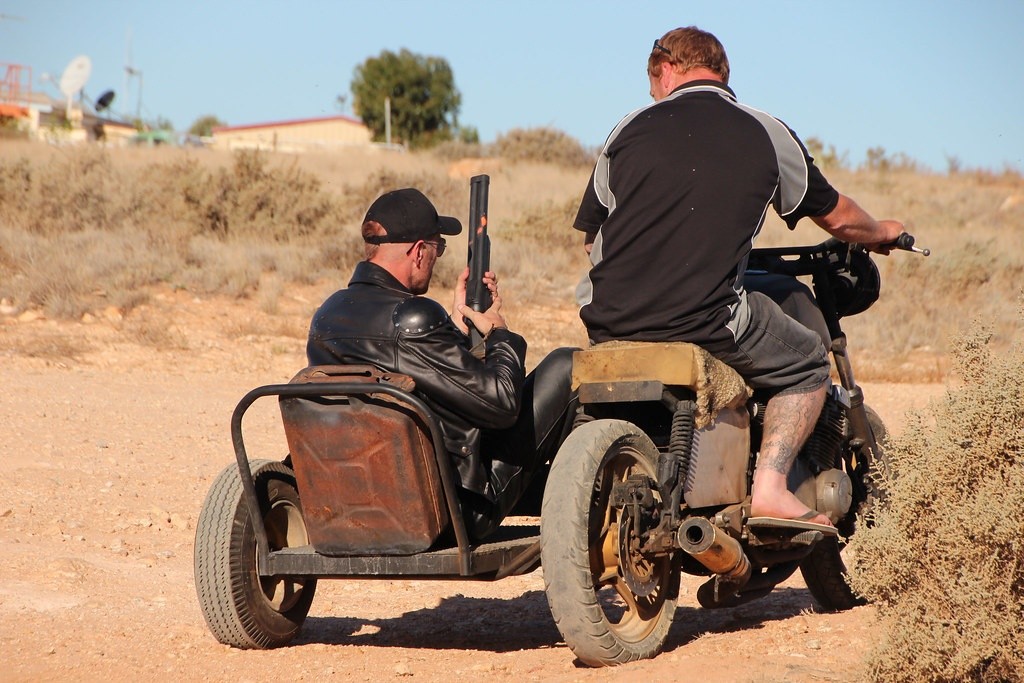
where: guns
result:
[459,172,493,365]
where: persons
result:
[575,28,907,532]
[307,188,584,551]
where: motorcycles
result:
[193,228,930,668]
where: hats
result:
[361,187,463,244]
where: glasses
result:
[406,237,447,257]
[653,39,671,56]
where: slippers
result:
[745,510,839,534]
